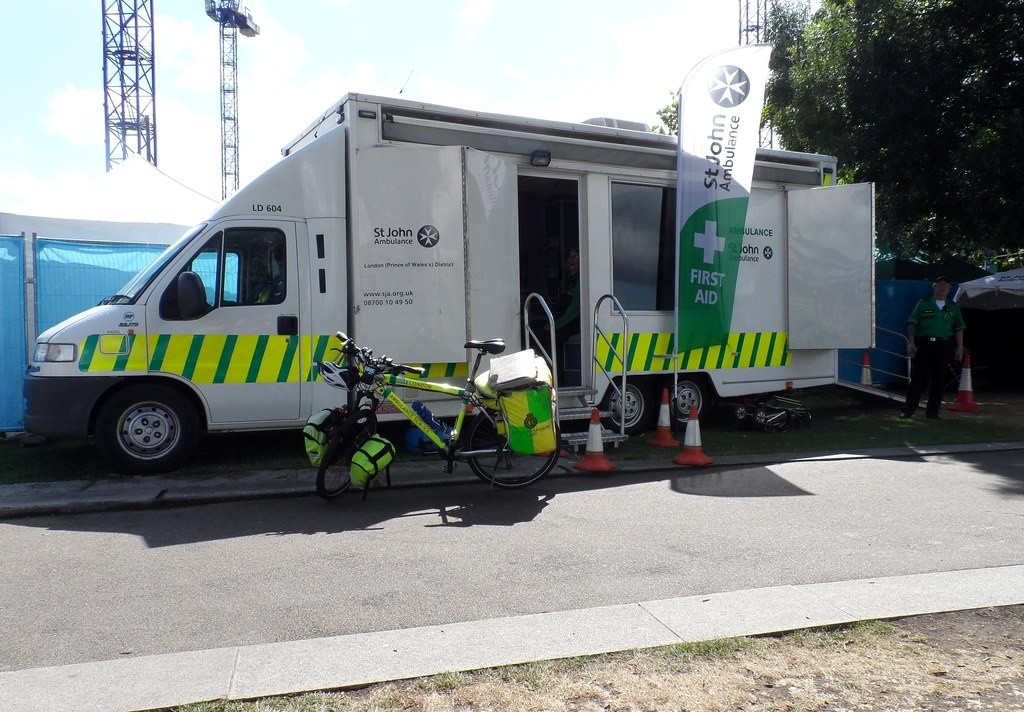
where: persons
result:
[899,276,964,421]
[532,247,580,360]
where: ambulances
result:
[16,88,879,478]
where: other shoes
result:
[899,410,912,418]
[927,414,940,420]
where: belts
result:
[920,337,950,342]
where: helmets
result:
[317,360,357,391]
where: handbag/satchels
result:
[502,385,556,455]
[303,406,339,467]
[350,434,396,490]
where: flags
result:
[679,44,774,355]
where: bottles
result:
[410,399,440,430]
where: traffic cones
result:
[859,351,874,397]
[946,353,982,413]
[672,404,713,466]
[646,388,682,448]
[576,408,616,472]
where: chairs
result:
[251,281,282,304]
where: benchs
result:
[563,333,581,372]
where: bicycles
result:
[301,331,562,501]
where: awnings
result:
[953,268,1024,302]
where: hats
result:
[935,276,951,283]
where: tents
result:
[1,154,238,437]
